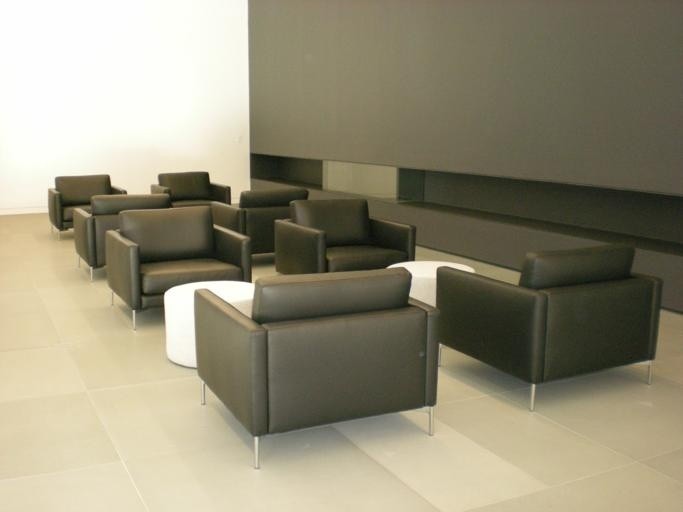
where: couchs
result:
[106,205,251,331]
[274,199,415,275]
[436,243,663,411]
[193,267,441,470]
[47,169,309,282]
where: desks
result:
[387,261,476,309]
[164,279,255,369]
